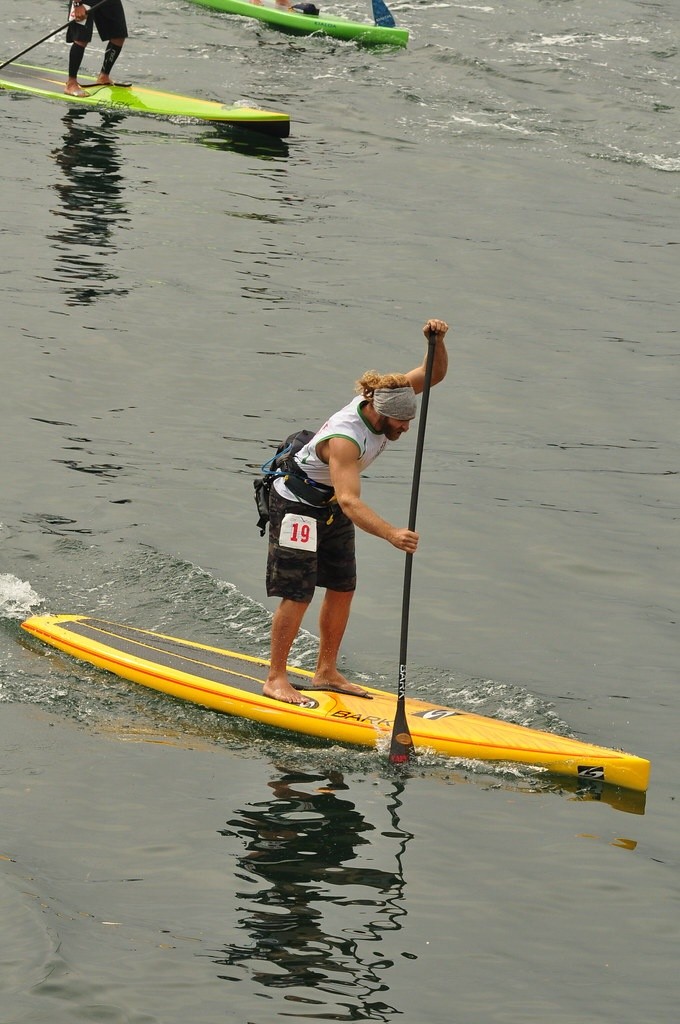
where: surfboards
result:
[16,608,653,793]
[0,59,292,138]
[187,0,410,48]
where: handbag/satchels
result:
[280,429,316,479]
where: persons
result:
[63,0,128,97]
[263,320,449,704]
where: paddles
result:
[0,0,108,69]
[388,329,440,756]
